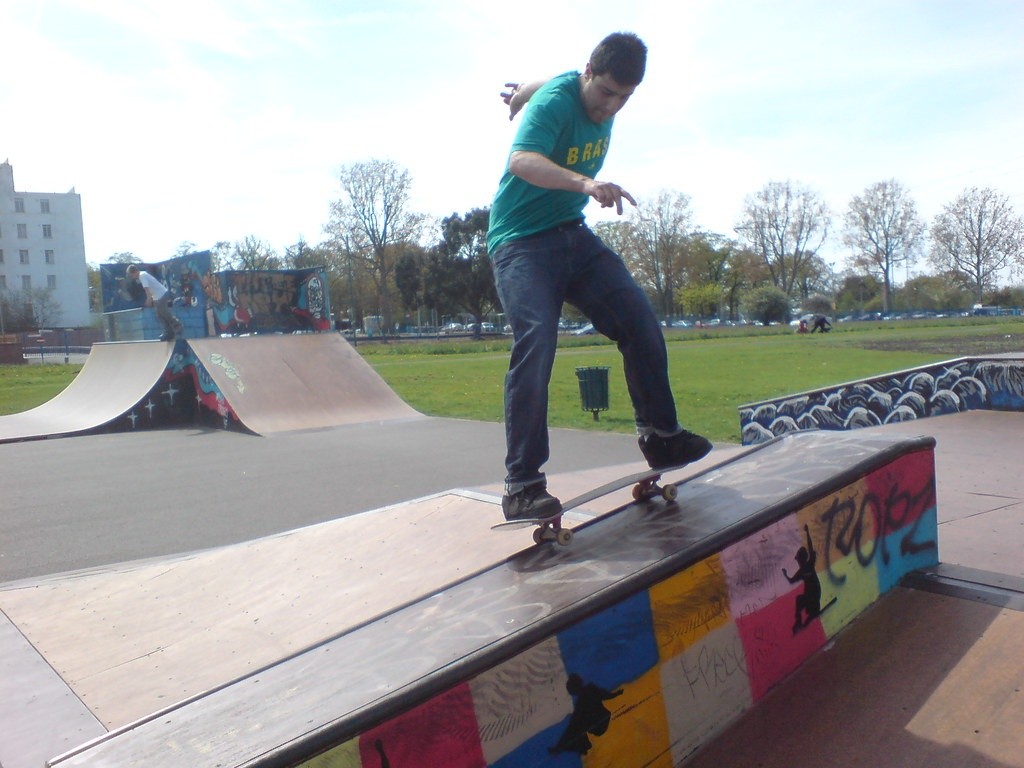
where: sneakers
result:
[638,430,713,468]
[501,484,563,521]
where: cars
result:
[342,322,512,334]
[658,304,1020,328]
[557,316,597,336]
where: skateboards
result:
[491,462,688,547]
[159,325,183,342]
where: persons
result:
[126,264,183,342]
[796,315,831,334]
[484,33,714,521]
